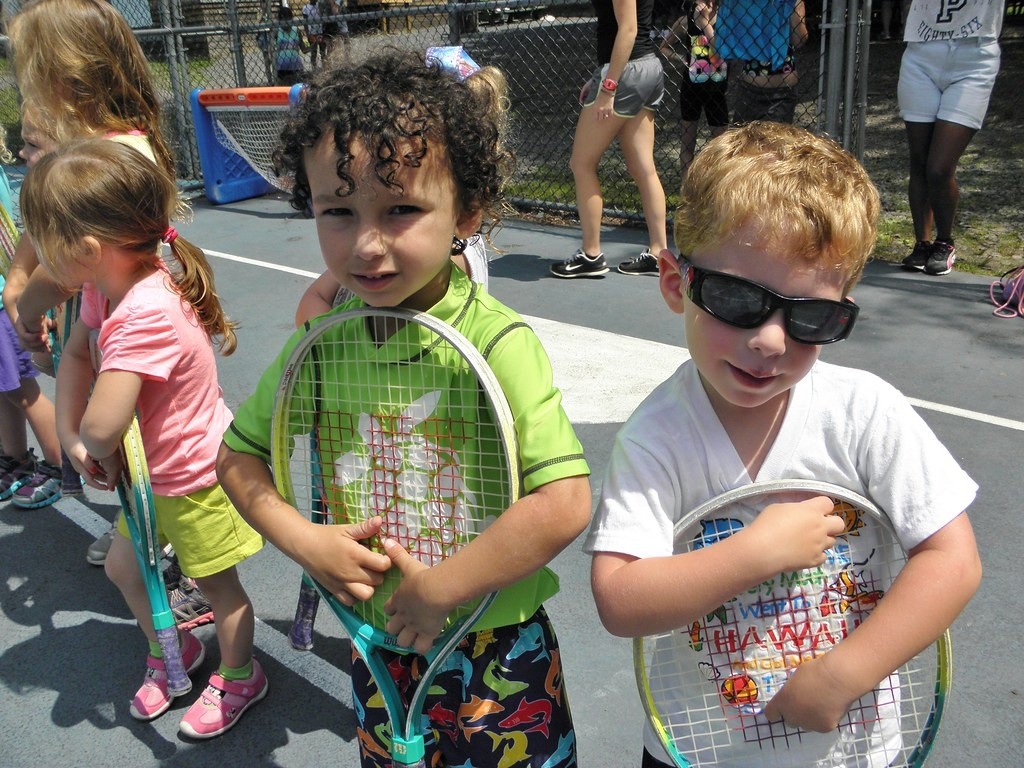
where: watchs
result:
[602,78,620,91]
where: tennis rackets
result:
[286,429,328,651]
[49,288,84,499]
[86,326,194,698]
[271,304,522,768]
[632,478,954,768]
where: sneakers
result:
[552,246,610,277]
[86,516,120,565]
[617,247,660,277]
[180,655,268,738]
[130,628,205,720]
[925,240,954,275]
[0,447,38,500]
[12,460,87,509]
[902,239,933,269]
[160,551,215,631]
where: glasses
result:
[676,252,860,344]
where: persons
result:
[896,0,1010,276]
[0,0,223,640]
[660,0,731,173]
[579,123,984,768]
[303,0,326,71]
[261,7,318,86]
[295,43,517,334]
[211,43,600,768]
[18,139,269,737]
[317,0,353,67]
[713,0,810,125]
[552,0,676,277]
